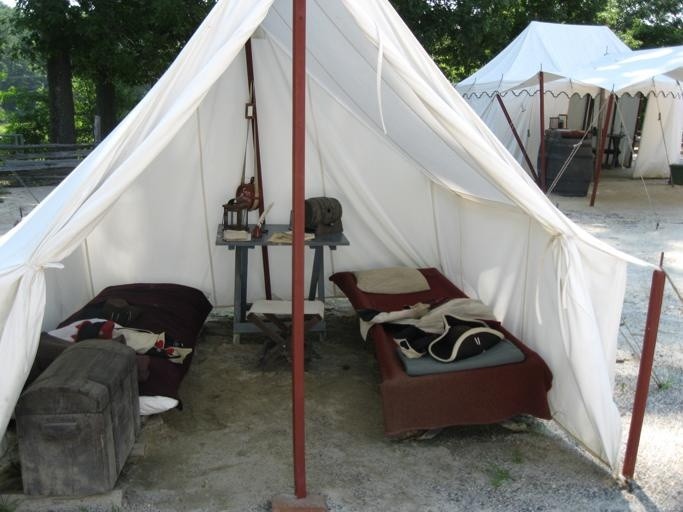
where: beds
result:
[329,267,553,436]
[48,282,215,412]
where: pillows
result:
[48,317,159,355]
[353,266,433,295]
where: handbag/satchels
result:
[306,197,343,235]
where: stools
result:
[246,300,325,373]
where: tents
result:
[0,0,654,512]
[453,19,683,180]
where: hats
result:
[429,314,504,363]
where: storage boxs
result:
[16,339,143,493]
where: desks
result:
[216,224,350,347]
